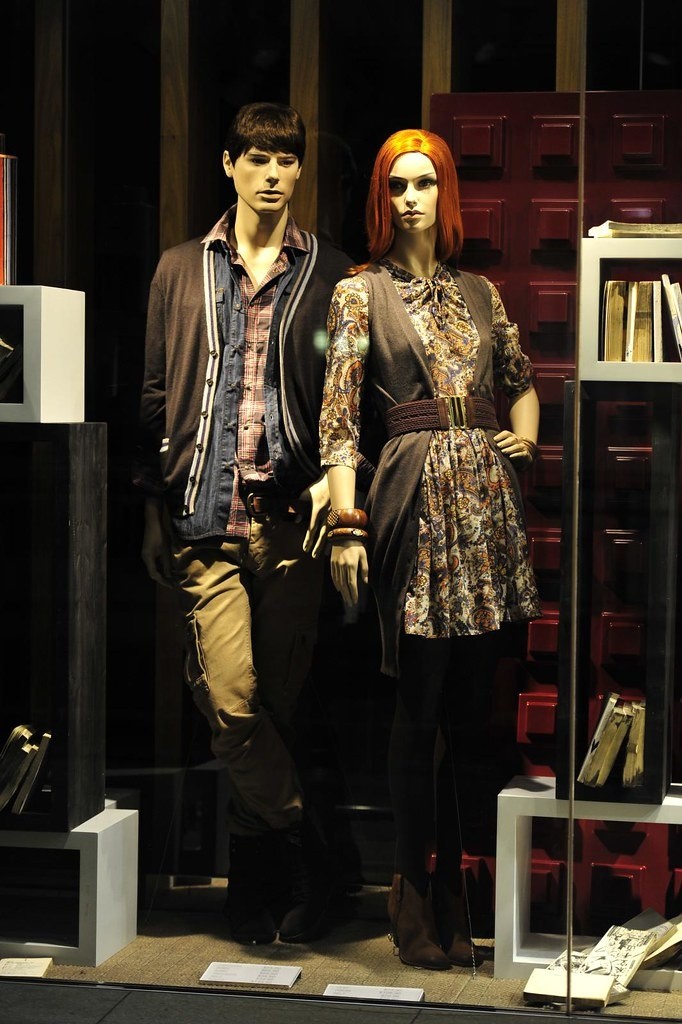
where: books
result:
[600,274,682,363]
[0,725,55,814]
[0,957,53,978]
[200,962,303,989]
[524,908,682,1011]
[576,692,645,790]
[588,220,682,238]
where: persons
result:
[128,103,358,944]
[317,129,538,971]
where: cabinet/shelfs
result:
[1,808,140,968]
[578,237,681,382]
[493,776,682,990]
[0,285,86,423]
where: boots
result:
[389,867,483,971]
[225,832,344,943]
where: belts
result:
[383,396,502,437]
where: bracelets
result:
[327,527,368,543]
[326,508,368,530]
